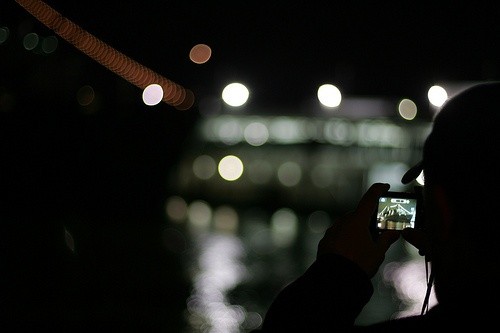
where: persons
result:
[250,82,500,333]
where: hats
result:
[402,82,500,184]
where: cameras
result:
[377,191,420,232]
